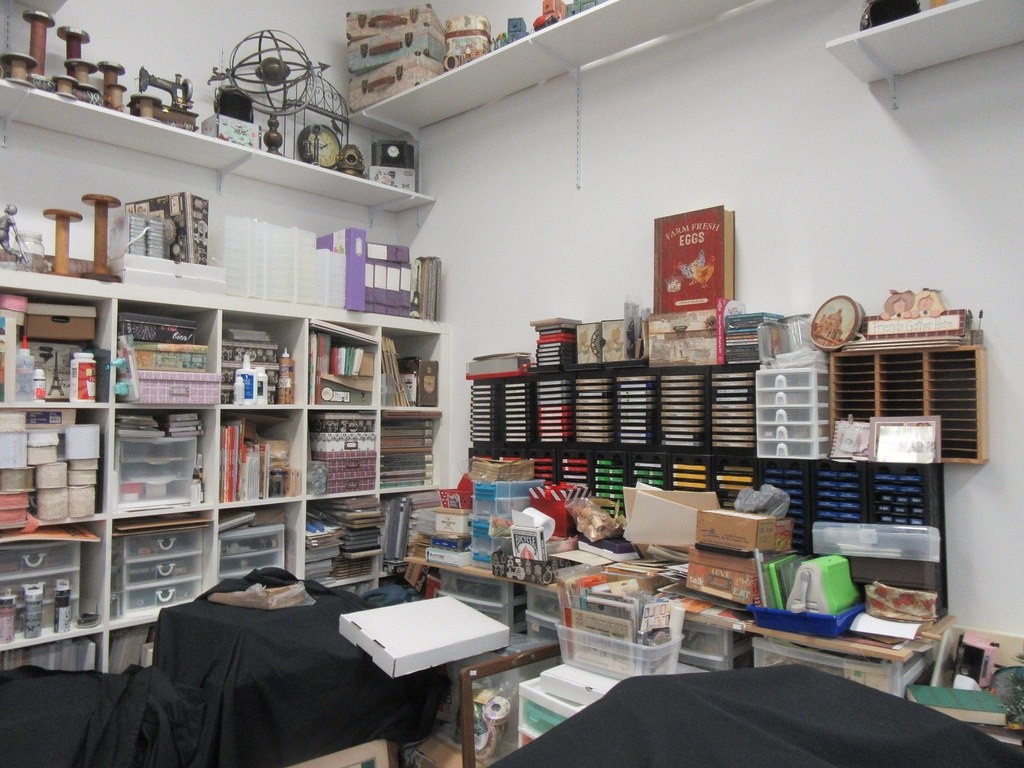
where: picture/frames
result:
[871,415,941,466]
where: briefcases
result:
[345,1,446,109]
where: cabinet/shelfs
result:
[827,345,990,463]
[1,267,446,674]
[0,0,1024,216]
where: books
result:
[653,206,737,312]
[114,409,289,504]
[905,683,1007,727]
[221,328,273,343]
[551,480,722,681]
[380,336,409,407]
[302,490,442,584]
[379,410,442,491]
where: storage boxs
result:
[115,526,203,621]
[200,114,262,151]
[645,310,718,367]
[24,300,97,339]
[1,407,77,431]
[367,241,414,315]
[371,163,416,192]
[339,462,1024,744]
[313,413,378,495]
[2,543,83,632]
[116,434,198,512]
[118,312,220,405]
[217,525,287,591]
[753,369,831,462]
[116,190,366,311]
